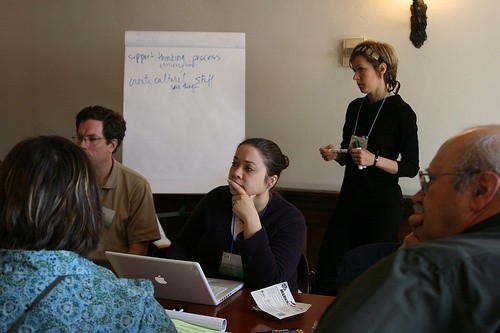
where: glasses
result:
[71,134,107,145]
[418,169,475,196]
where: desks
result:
[155,290,337,333]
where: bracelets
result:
[373,155,378,167]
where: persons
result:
[308,124,500,333]
[165,138,307,294]
[72,105,162,258]
[318,38,420,297]
[0,136,178,333]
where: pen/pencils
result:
[173,307,177,311]
[180,304,187,311]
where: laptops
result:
[105,251,244,306]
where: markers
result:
[330,149,349,153]
[351,140,367,170]
